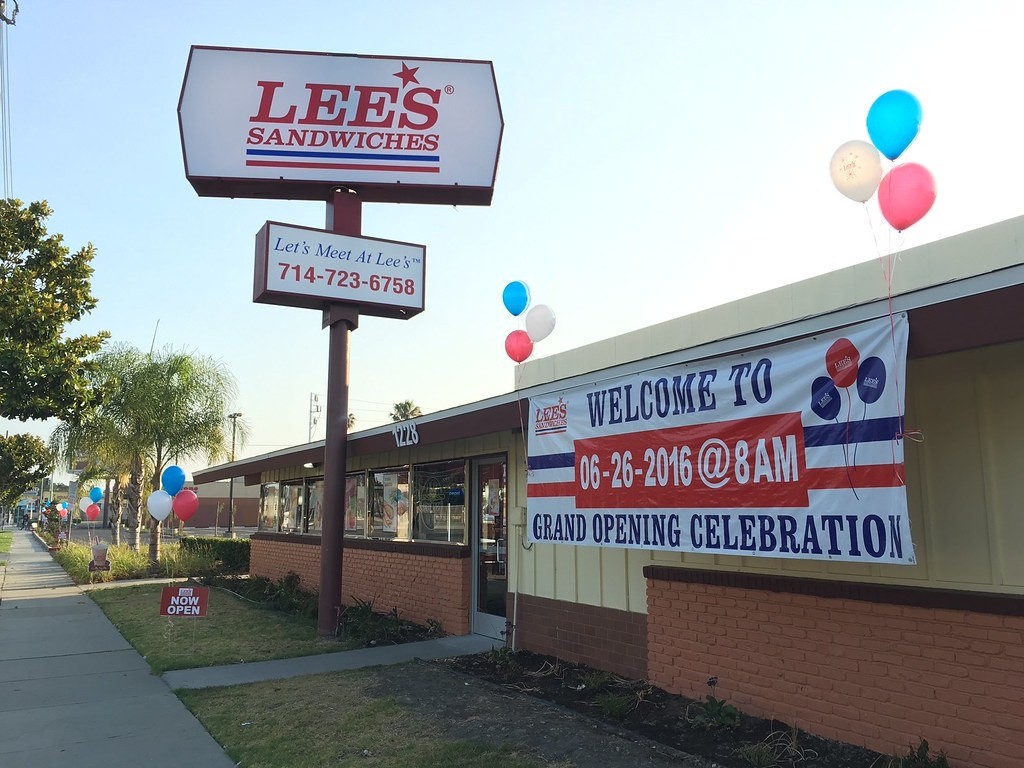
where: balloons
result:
[86,504,100,520]
[867,89,923,161]
[81,497,93,512]
[162,466,185,496]
[526,304,556,342]
[831,140,881,202]
[147,490,172,522]
[46,502,50,506]
[505,330,533,363]
[503,280,528,316]
[90,487,102,503]
[878,162,937,233]
[56,502,68,517]
[173,490,199,521]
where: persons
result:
[21,514,28,530]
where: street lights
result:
[225,412,244,533]
